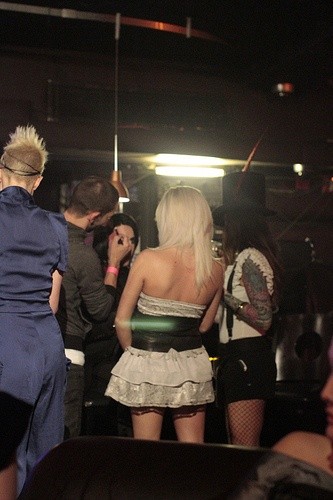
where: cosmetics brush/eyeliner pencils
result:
[115,227,123,244]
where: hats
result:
[212,172,275,227]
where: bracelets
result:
[236,302,248,320]
[106,266,118,277]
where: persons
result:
[80,213,139,437]
[274,330,333,472]
[104,186,225,443]
[212,172,280,447]
[1,124,70,500]
[57,179,131,439]
